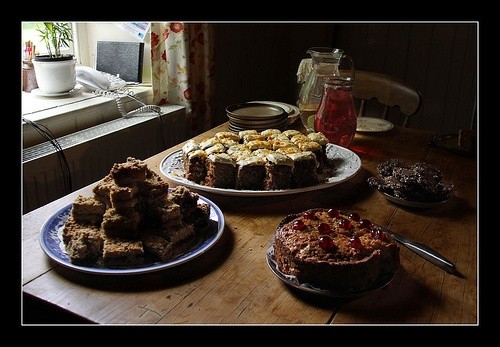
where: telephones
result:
[75,65,127,93]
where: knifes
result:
[375,223,456,272]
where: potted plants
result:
[32,23,77,93]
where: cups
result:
[315,77,357,147]
[23,60,38,92]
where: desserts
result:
[63,158,210,263]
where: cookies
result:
[367,159,455,202]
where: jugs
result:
[299,47,355,133]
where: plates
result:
[356,117,393,132]
[42,188,225,275]
[380,191,452,208]
[266,256,400,298]
[226,100,300,135]
[159,142,361,196]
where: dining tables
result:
[23,120,477,324]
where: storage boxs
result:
[96,40,145,83]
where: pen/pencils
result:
[24,40,38,61]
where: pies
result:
[183,130,328,189]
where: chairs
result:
[339,69,423,127]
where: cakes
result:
[273,209,397,289]
